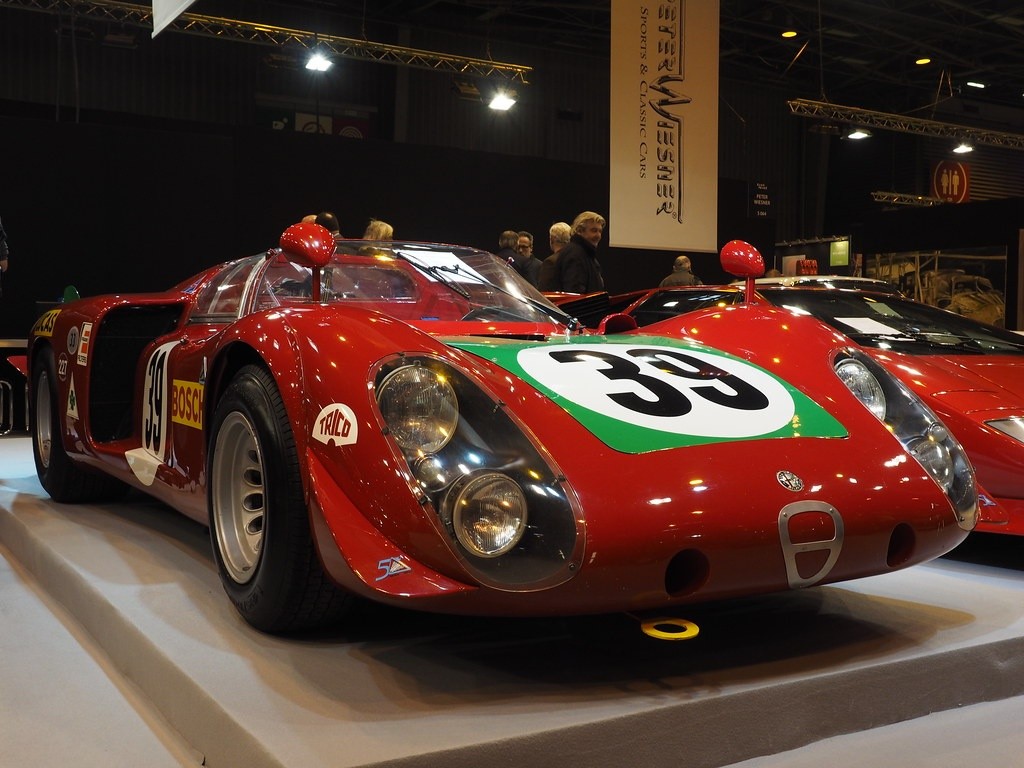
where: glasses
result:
[519,245,531,248]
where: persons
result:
[516,231,544,266]
[0,217,10,273]
[559,211,606,293]
[538,222,573,286]
[494,230,536,289]
[362,220,394,241]
[658,256,703,287]
[315,212,345,239]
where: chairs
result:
[7,356,29,431]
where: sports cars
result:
[536,274,1024,537]
[26,223,978,642]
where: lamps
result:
[472,69,517,111]
